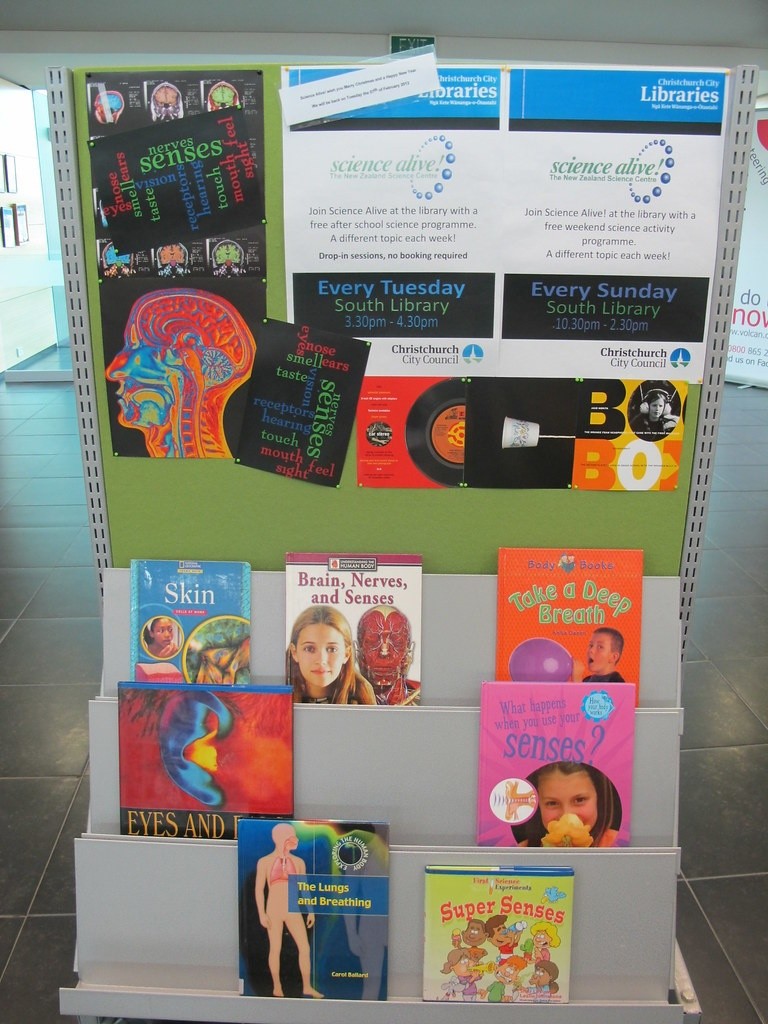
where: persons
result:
[286,605,377,704]
[582,627,626,683]
[630,389,677,441]
[518,761,619,848]
[144,618,179,658]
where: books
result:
[495,547,643,708]
[129,559,251,685]
[285,551,422,706]
[117,681,295,840]
[237,817,390,1001]
[423,864,574,1003]
[476,681,635,848]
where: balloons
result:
[509,638,573,682]
